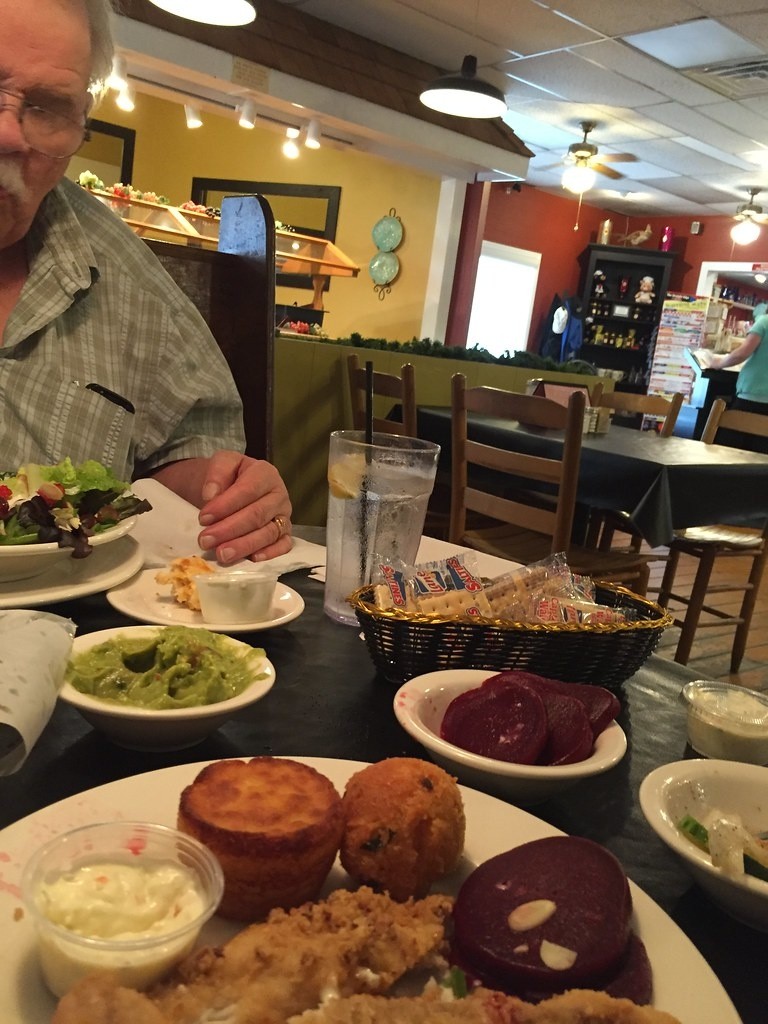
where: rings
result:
[272,517,285,541]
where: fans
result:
[531,120,640,180]
[732,188,768,226]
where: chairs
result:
[628,398,768,674]
[448,373,659,599]
[346,353,504,541]
[590,381,685,549]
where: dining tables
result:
[0,524,768,1024]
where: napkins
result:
[308,535,525,584]
[130,479,327,576]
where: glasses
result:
[0,89,87,159]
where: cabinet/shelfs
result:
[576,244,679,429]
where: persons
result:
[708,314,768,453]
[0,0,294,565]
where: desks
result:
[381,403,768,549]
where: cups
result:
[323,429,440,629]
[678,679,768,769]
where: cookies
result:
[371,553,636,625]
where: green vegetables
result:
[0,457,152,560]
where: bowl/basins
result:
[0,514,138,582]
[20,824,226,999]
[638,758,768,932]
[57,625,276,751]
[392,668,628,803]
[192,571,281,624]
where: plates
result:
[369,251,399,286]
[106,568,305,632]
[372,216,403,252]
[0,535,145,607]
[0,755,740,1023]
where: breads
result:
[175,755,345,923]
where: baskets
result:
[343,574,675,690]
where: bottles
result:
[583,369,615,435]
[661,226,673,251]
[589,302,641,320]
[596,218,612,246]
[584,325,632,349]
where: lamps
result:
[149,0,257,26]
[116,84,136,112]
[561,159,596,195]
[419,53,507,119]
[183,103,204,129]
[729,217,761,246]
[107,56,128,90]
[301,119,322,150]
[235,97,258,130]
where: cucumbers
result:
[678,815,767,883]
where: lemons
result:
[328,463,361,500]
